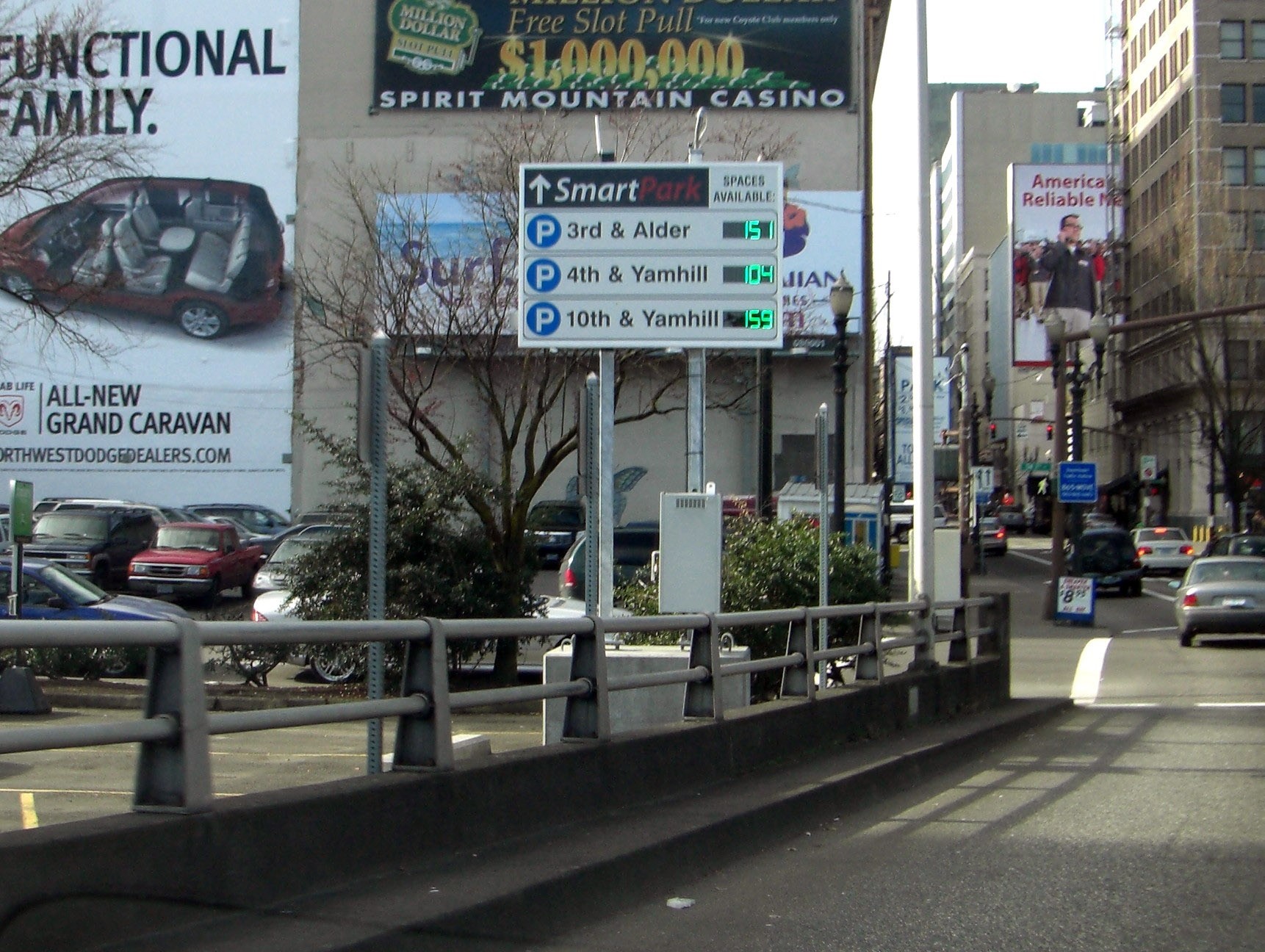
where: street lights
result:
[828,271,854,546]
[954,363,995,577]
[1043,302,1111,578]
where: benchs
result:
[183,214,250,293]
[183,195,241,232]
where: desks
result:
[158,226,195,268]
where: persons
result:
[1011,238,1106,325]
[1037,213,1098,360]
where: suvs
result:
[523,500,585,571]
[557,521,660,610]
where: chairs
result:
[72,194,172,297]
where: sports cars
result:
[0,175,287,341]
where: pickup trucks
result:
[127,521,264,610]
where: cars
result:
[0,555,192,679]
[0,494,368,686]
[888,494,1027,558]
[1060,526,1144,599]
[1199,530,1265,558]
[1032,502,1119,533]
[1127,526,1198,578]
[1168,555,1265,648]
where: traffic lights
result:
[1026,475,1051,497]
[1046,424,1053,440]
[990,423,997,438]
[1145,483,1160,496]
